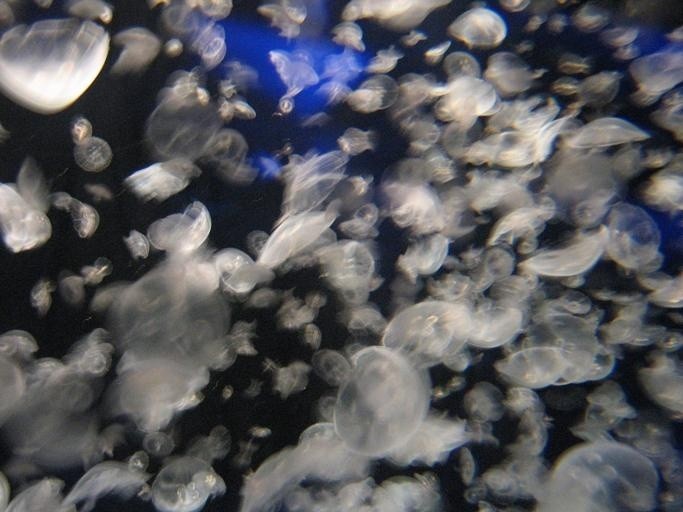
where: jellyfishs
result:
[1,0,682,512]
